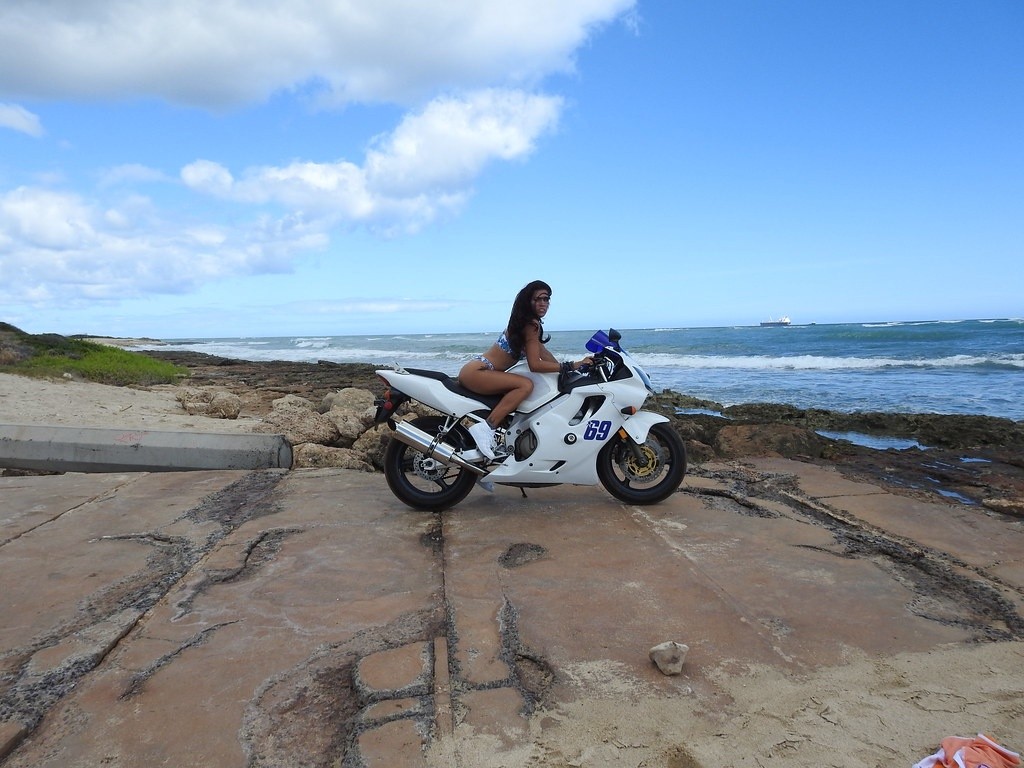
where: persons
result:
[458,280,592,492]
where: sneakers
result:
[468,419,495,460]
[476,474,495,492]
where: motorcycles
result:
[372,326,688,512]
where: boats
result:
[760,315,791,327]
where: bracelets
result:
[560,361,574,372]
